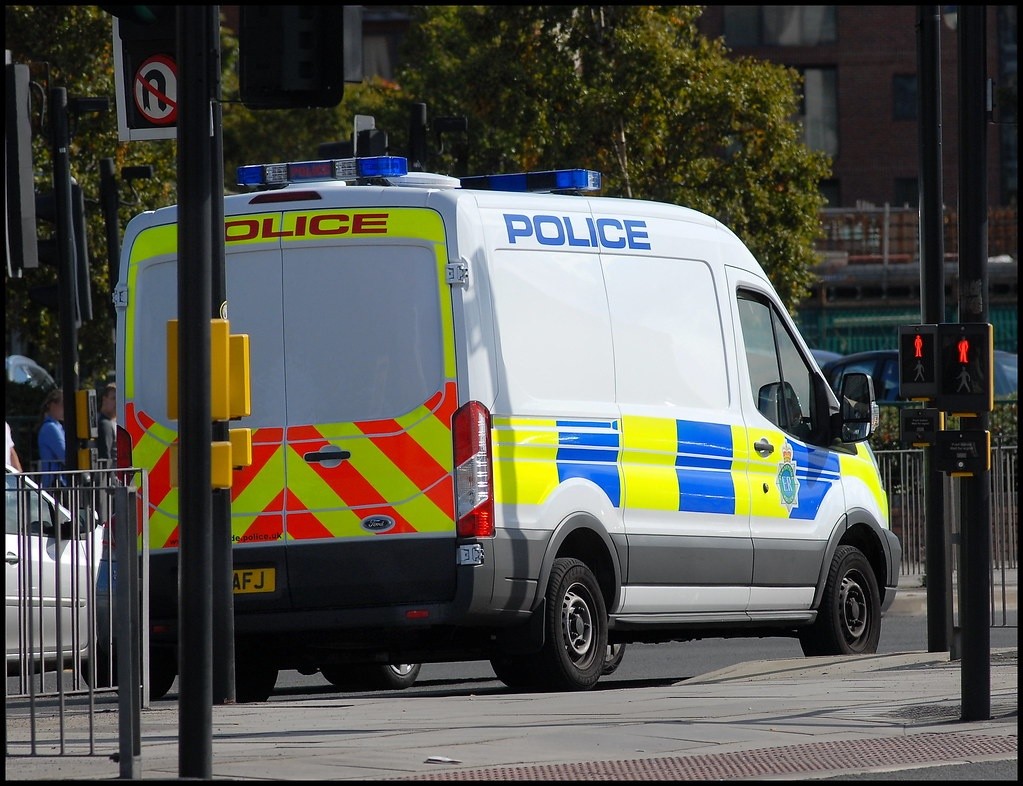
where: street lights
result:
[409,116,469,171]
[49,93,110,508]
[102,165,153,324]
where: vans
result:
[113,159,904,694]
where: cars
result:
[821,348,1018,408]
[6,465,107,691]
[747,349,898,403]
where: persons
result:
[35,383,116,527]
[5,421,23,471]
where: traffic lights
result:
[315,116,376,158]
[938,324,995,414]
[898,324,938,403]
[75,389,99,439]
[101,2,176,52]
